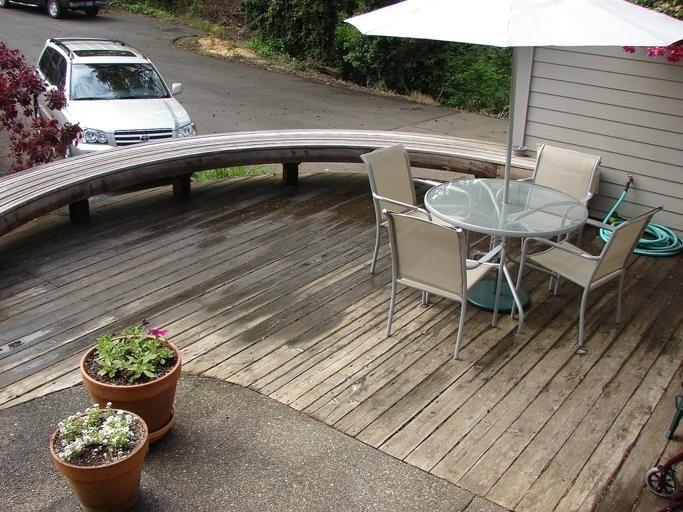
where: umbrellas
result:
[341,1,682,295]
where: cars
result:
[0,1,114,20]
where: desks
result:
[423,178,590,312]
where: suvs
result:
[31,35,195,163]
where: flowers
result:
[53,401,137,466]
[93,316,175,384]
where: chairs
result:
[499,144,602,291]
[381,207,524,362]
[360,144,455,305]
[511,205,665,354]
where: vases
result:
[79,334,182,445]
[50,408,149,511]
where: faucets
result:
[625,174,633,183]
